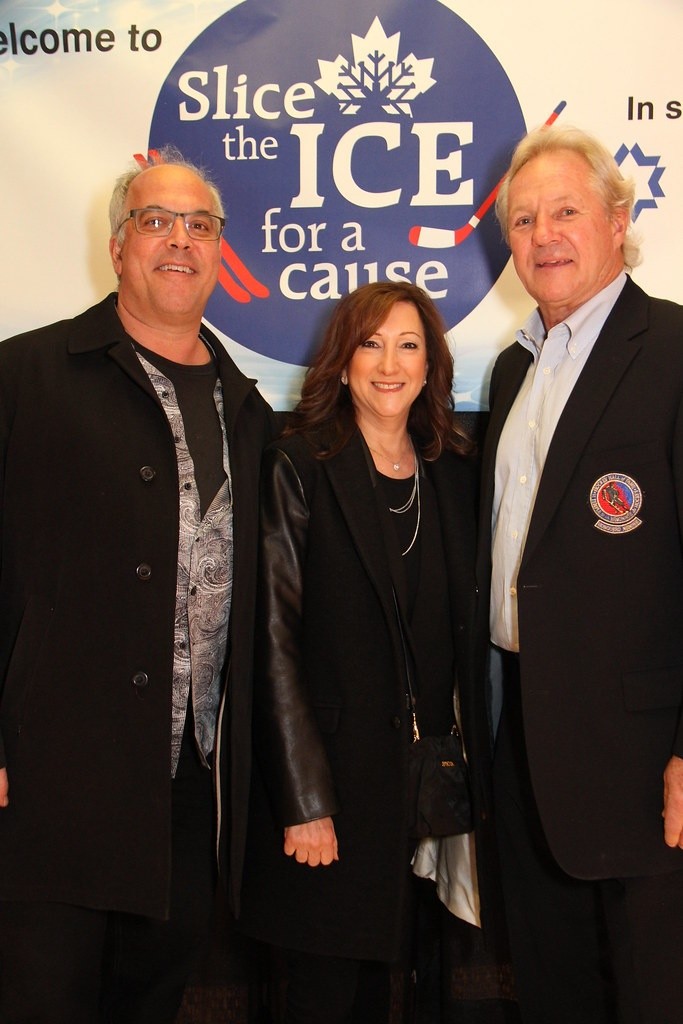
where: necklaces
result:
[368,431,421,558]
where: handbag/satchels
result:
[406,737,472,837]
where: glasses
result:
[117,207,226,241]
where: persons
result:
[258,281,480,1024]
[0,156,275,1024]
[478,130,683,1024]
